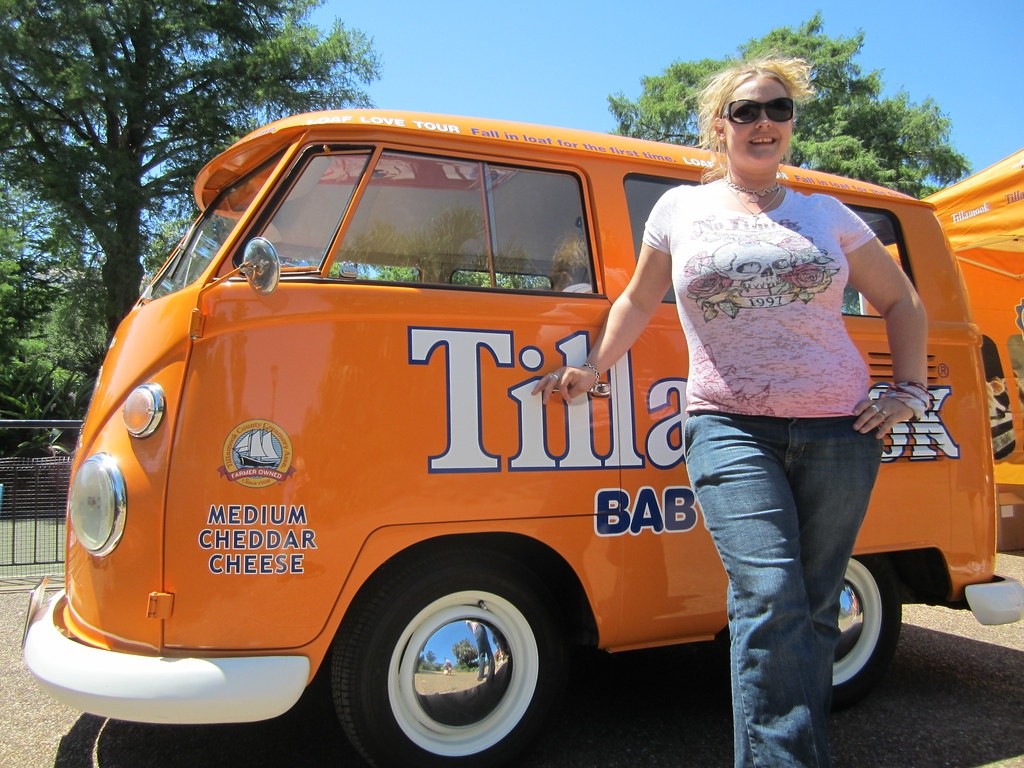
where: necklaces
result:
[724,176,782,221]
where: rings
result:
[881,410,886,415]
[550,373,559,380]
[871,404,879,413]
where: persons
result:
[529,55,940,768]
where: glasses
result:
[720,97,794,124]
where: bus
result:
[21,109,1022,768]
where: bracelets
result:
[583,362,600,391]
